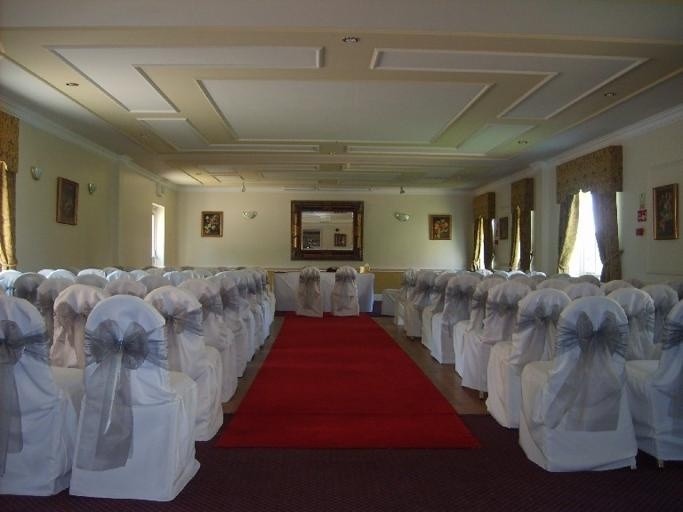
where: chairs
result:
[294,265,360,320]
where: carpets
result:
[213,306,484,450]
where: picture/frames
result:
[652,183,679,240]
[429,213,452,241]
[201,210,224,238]
[55,176,80,226]
